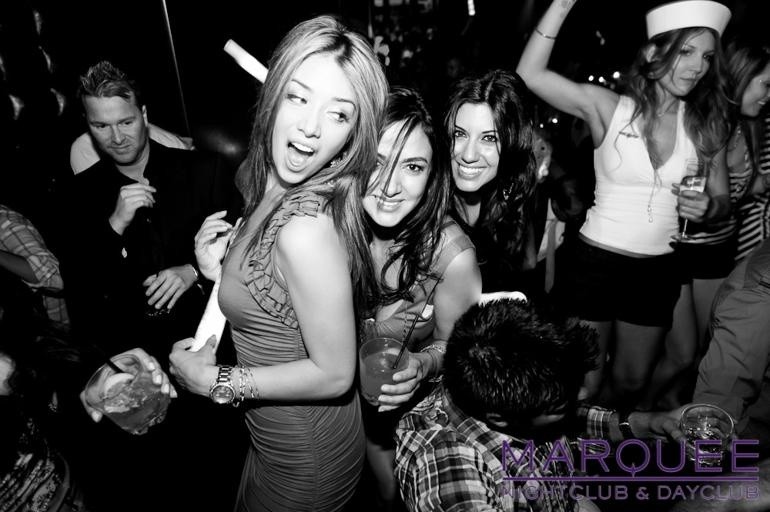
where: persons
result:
[0,1,769,512]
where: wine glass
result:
[670,157,710,243]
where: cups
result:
[358,338,411,408]
[679,404,736,466]
[83,354,173,436]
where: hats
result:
[646,1,732,39]
